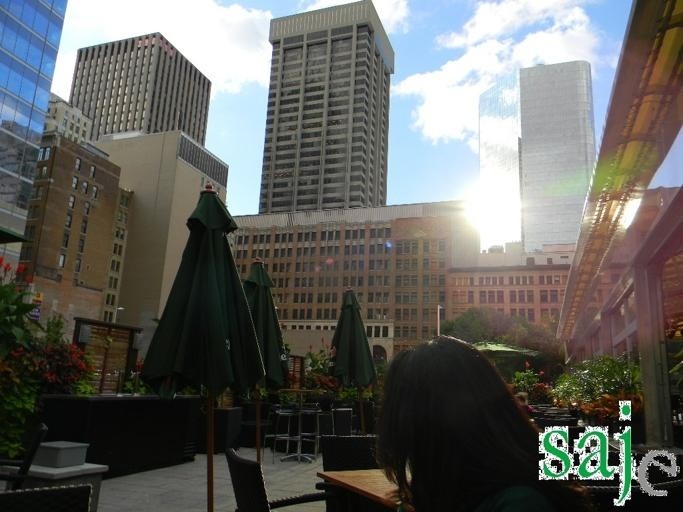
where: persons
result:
[517,391,534,418]
[376,335,593,511]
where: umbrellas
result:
[242,254,289,463]
[139,181,264,512]
[331,287,376,433]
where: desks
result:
[317,468,401,512]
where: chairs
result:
[532,403,683,511]
[227,408,380,512]
[0,420,92,512]
